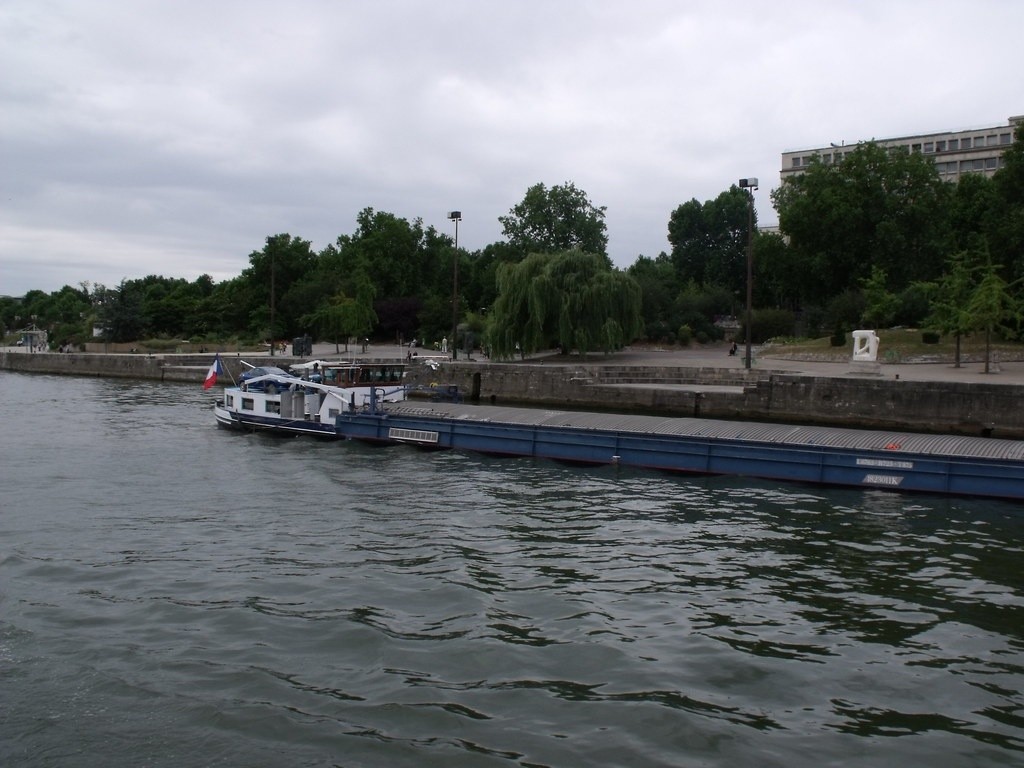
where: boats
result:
[288,360,408,404]
[214,367,349,440]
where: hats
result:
[443,336,446,338]
[313,363,319,367]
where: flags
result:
[204,353,225,388]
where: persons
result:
[442,335,448,355]
[729,341,738,357]
[478,341,521,358]
[406,350,418,362]
[359,369,397,382]
[58,344,71,352]
[278,342,287,354]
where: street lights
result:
[448,211,461,358]
[265,237,277,356]
[740,178,757,367]
